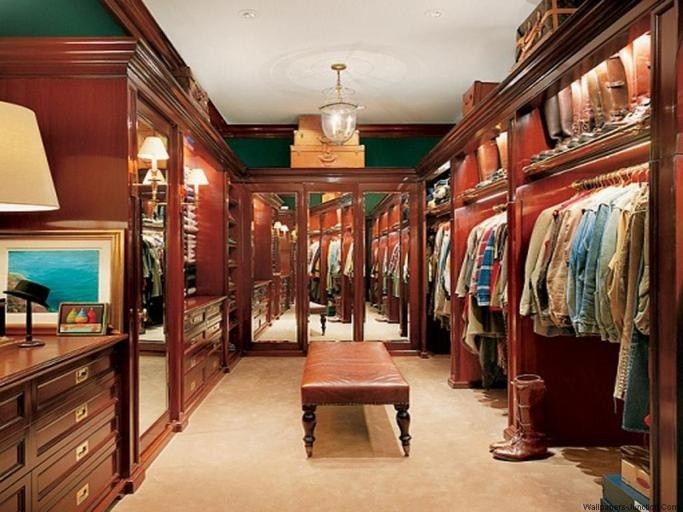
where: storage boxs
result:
[462,80,500,116]
[516,1,584,63]
[289,114,365,168]
[172,68,209,114]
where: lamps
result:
[183,167,208,202]
[317,64,358,146]
[0,101,60,214]
[136,136,171,207]
[272,220,289,240]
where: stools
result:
[300,341,412,458]
[309,301,327,336]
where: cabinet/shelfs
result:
[252,275,289,339]
[308,194,351,322]
[368,193,410,323]
[130,80,245,493]
[423,0,657,510]
[0,330,127,512]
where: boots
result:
[375,297,388,322]
[328,297,342,322]
[489,373,542,450]
[493,383,549,461]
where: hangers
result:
[569,160,650,194]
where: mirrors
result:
[250,192,412,345]
[136,114,169,440]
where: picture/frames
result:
[0,228,125,337]
